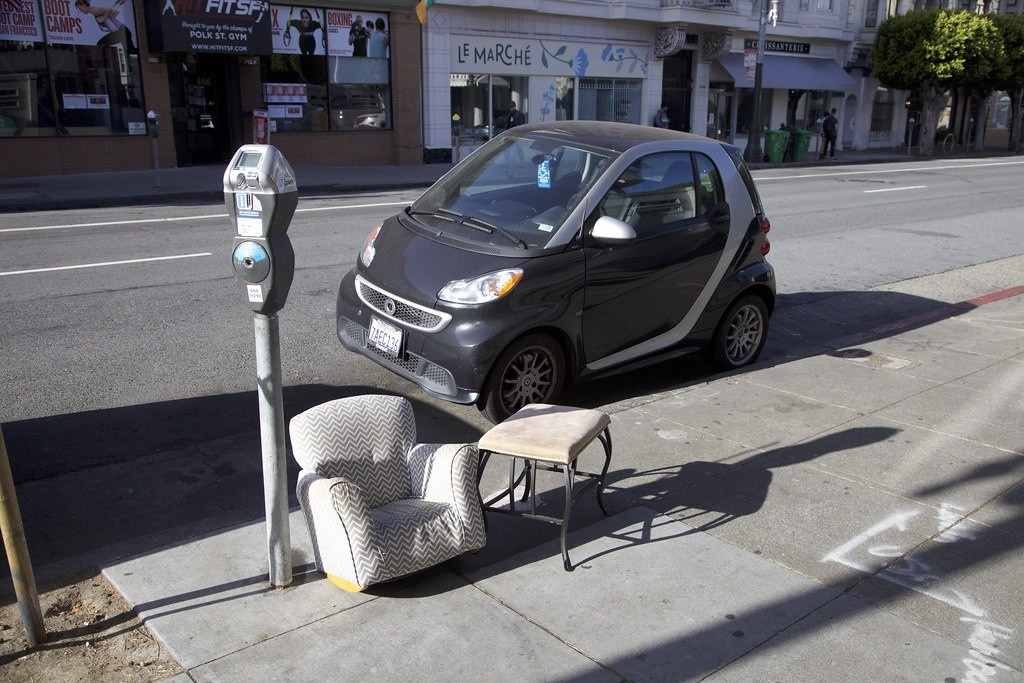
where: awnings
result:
[717,52,860,92]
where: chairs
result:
[565,159,641,228]
[627,161,706,232]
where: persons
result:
[284,9,326,55]
[503,102,525,130]
[556,97,567,120]
[349,15,389,58]
[817,108,838,160]
[654,103,671,129]
[75,0,135,49]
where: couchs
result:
[289,395,485,593]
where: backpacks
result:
[823,118,834,133]
[654,111,662,127]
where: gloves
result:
[352,22,359,36]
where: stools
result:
[476,401,613,572]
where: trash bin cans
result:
[792,129,812,162]
[763,129,791,164]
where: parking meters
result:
[223,142,299,589]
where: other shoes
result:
[818,158,826,161]
[831,157,838,160]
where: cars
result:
[336,121,777,427]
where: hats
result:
[508,101,516,107]
